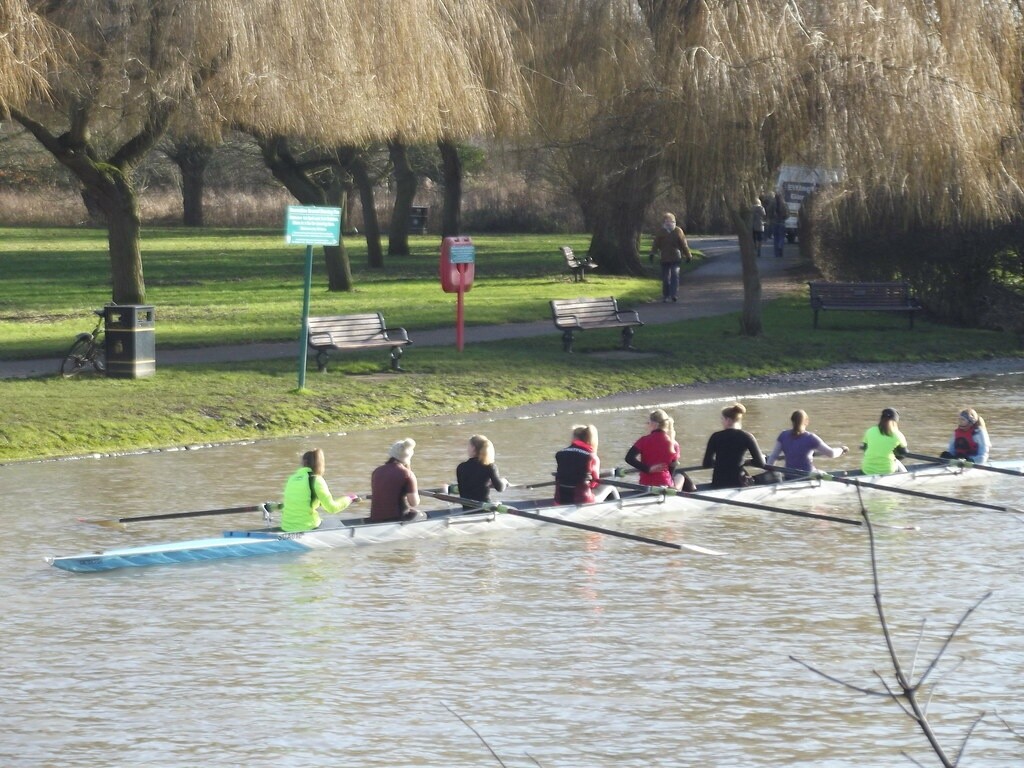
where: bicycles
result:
[60,303,114,379]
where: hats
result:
[882,408,900,422]
[390,438,416,464]
[662,212,675,222]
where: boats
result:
[45,460,1023,574]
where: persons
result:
[860,409,909,475]
[649,212,692,303]
[624,409,697,492]
[940,408,990,464]
[553,425,620,506]
[751,192,789,258]
[282,449,359,531]
[456,435,509,511]
[703,403,766,489]
[769,409,850,480]
[371,439,428,521]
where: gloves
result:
[649,254,654,262]
[686,257,692,263]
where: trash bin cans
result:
[408,206,428,235]
[104,304,155,377]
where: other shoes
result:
[663,296,680,303]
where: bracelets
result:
[841,447,845,451]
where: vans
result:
[778,164,821,243]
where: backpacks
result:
[776,203,789,219]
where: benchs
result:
[810,283,922,328]
[560,246,597,280]
[310,312,411,373]
[552,298,643,351]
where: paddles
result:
[858,445,1024,476]
[360,482,509,500]
[416,488,722,555]
[79,494,361,528]
[550,472,919,533]
[681,450,845,471]
[745,461,1008,512]
[510,468,639,488]
